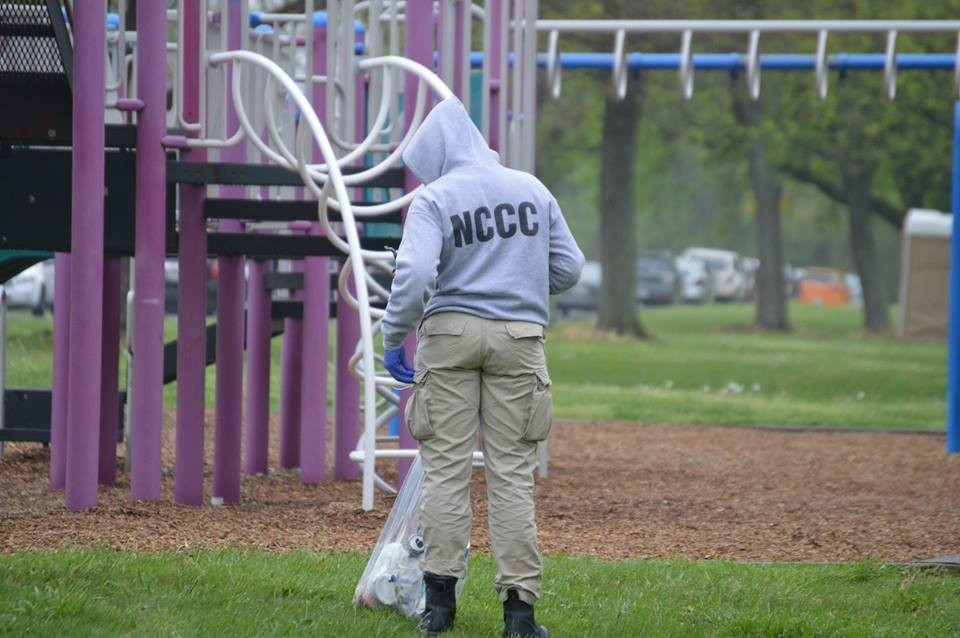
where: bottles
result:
[394,557,421,616]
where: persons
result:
[380,95,586,638]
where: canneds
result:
[406,534,425,558]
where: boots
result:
[418,571,459,636]
[502,587,549,638]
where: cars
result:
[4,252,247,314]
[556,246,863,310]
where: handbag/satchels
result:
[353,449,469,624]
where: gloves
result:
[384,346,416,383]
